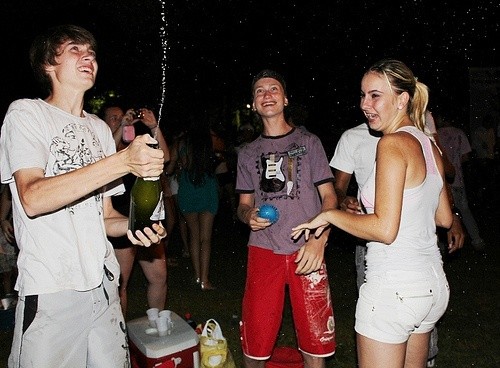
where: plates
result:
[144,328,170,335]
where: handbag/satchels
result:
[199,319,236,368]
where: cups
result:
[159,310,170,325]
[147,308,159,328]
[156,318,168,336]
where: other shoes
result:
[192,273,216,291]
[471,242,487,258]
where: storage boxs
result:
[126,310,199,368]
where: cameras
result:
[133,110,145,119]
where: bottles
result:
[129,142,161,239]
